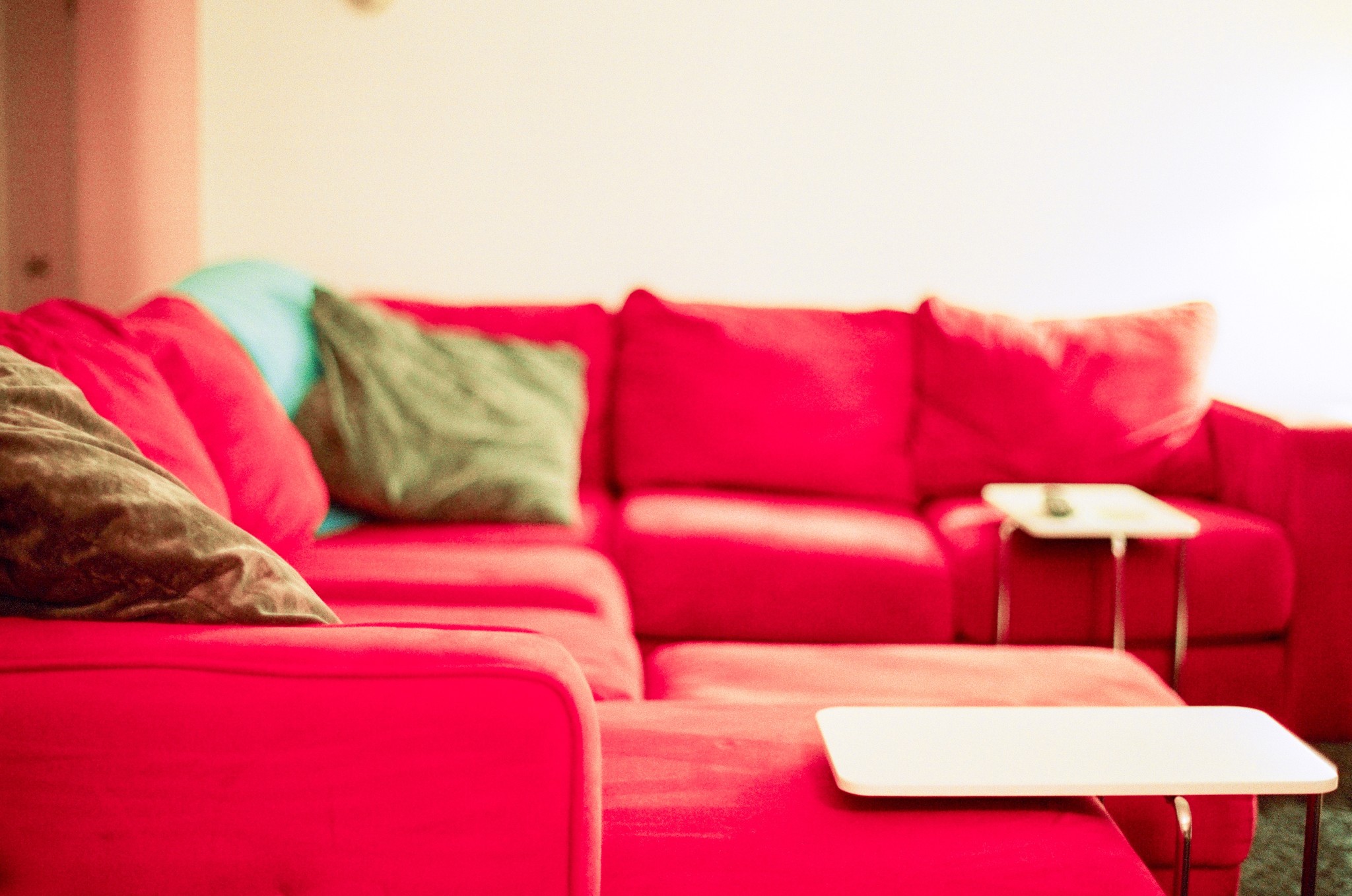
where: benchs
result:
[648,643,1257,895]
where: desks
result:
[818,705,1340,896]
[982,482,1201,692]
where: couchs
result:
[0,255,1352,896]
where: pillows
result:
[0,346,342,628]
[291,287,588,530]
